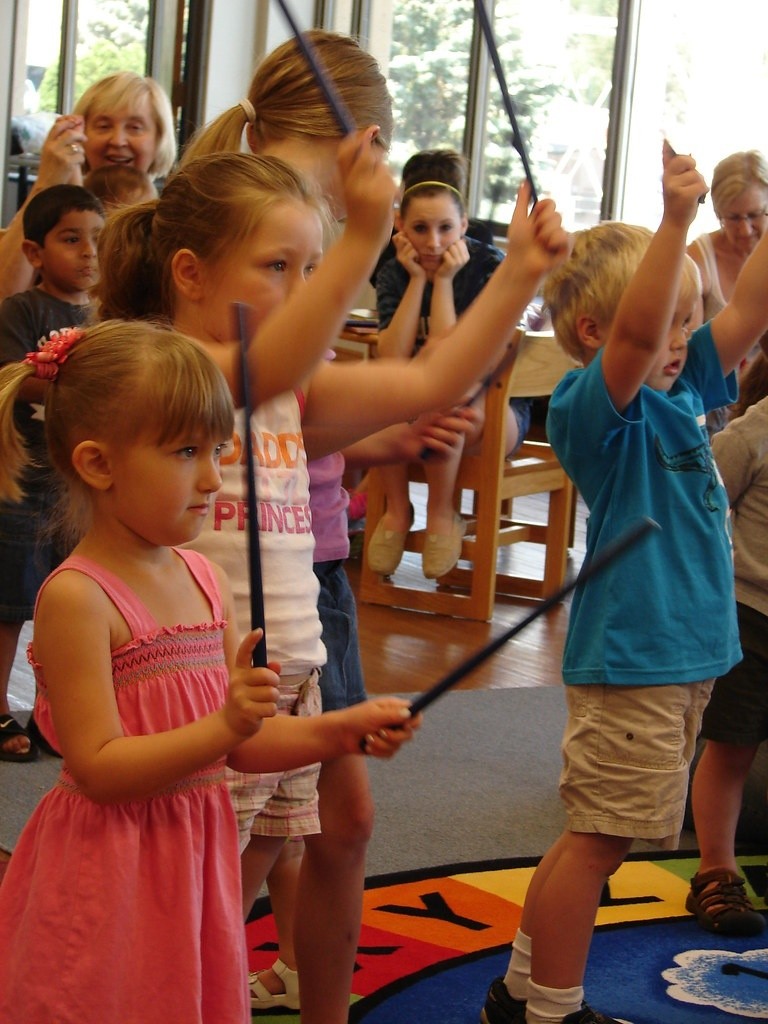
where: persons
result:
[481,149,768,1024]
[0,319,427,1022]
[0,33,510,1023]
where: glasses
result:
[719,205,768,229]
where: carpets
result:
[0,683,768,1024]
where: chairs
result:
[358,324,583,622]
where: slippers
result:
[24,716,63,758]
[0,713,38,763]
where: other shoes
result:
[482,981,529,1024]
[369,501,415,574]
[424,511,467,578]
[558,1000,634,1024]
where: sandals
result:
[249,956,300,1009]
[686,871,765,933]
